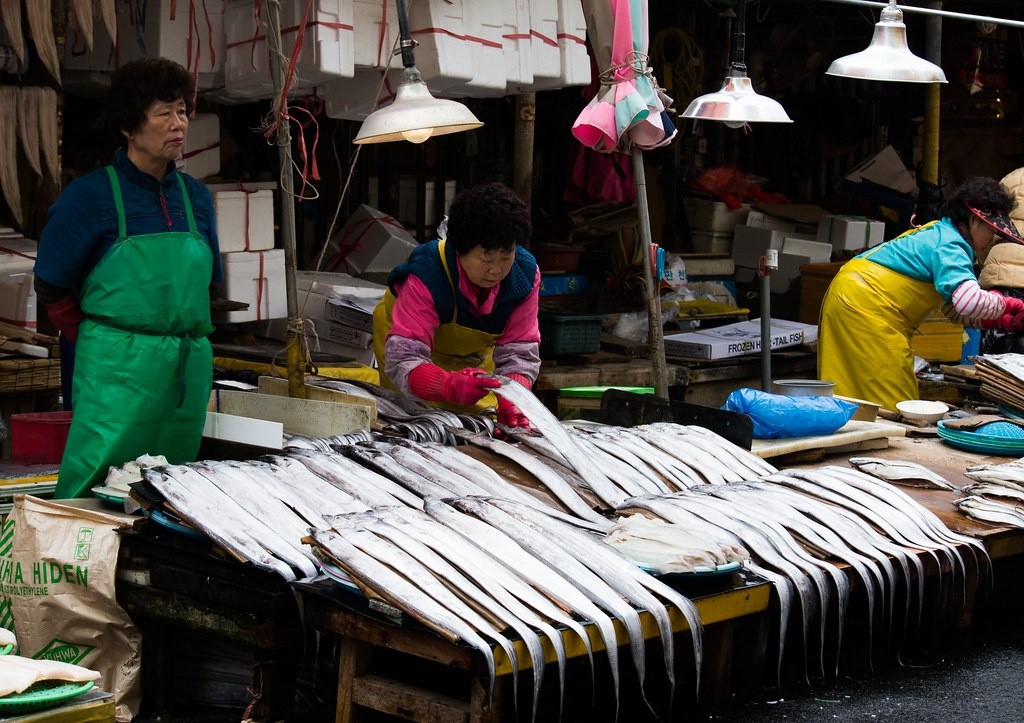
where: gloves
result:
[492,372,533,444]
[408,361,502,407]
[982,288,1024,334]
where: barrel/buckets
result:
[833,394,882,422]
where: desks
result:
[86,407,1024,723]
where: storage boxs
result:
[298,272,386,370]
[817,212,886,252]
[908,321,964,363]
[539,313,609,354]
[211,189,275,252]
[11,412,72,464]
[689,230,732,254]
[217,249,289,323]
[779,237,833,274]
[662,317,818,359]
[0,252,38,333]
[685,196,753,234]
[66,0,591,121]
[333,205,419,271]
[731,224,815,268]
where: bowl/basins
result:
[896,400,949,424]
[773,380,836,398]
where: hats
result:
[971,204,1024,248]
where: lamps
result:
[351,1,483,145]
[677,0,794,127]
[825,1,949,85]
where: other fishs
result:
[139,374,1024,723]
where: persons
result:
[978,167,1024,355]
[31,55,227,500]
[816,175,1024,410]
[371,184,542,438]
